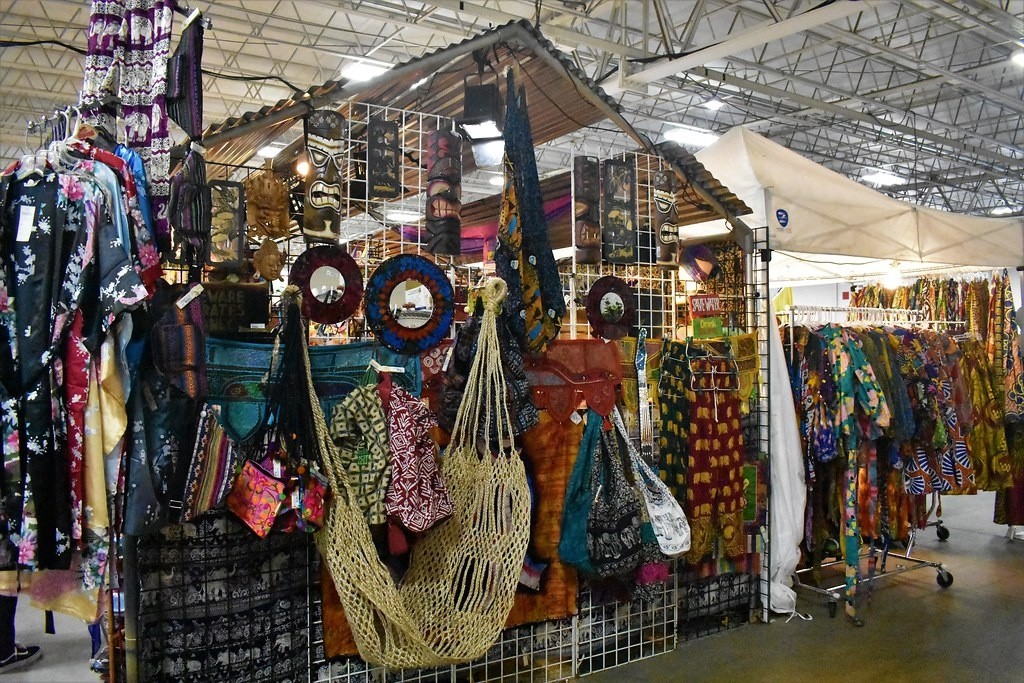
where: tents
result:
[693,124,1023,294]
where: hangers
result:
[854,267,1010,294]
[11,105,116,183]
[778,304,984,344]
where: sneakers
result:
[0,645,42,673]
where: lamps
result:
[462,58,510,168]
[703,94,724,111]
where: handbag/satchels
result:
[225,446,328,540]
[557,405,691,602]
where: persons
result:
[0,593,43,673]
[254,236,285,281]
